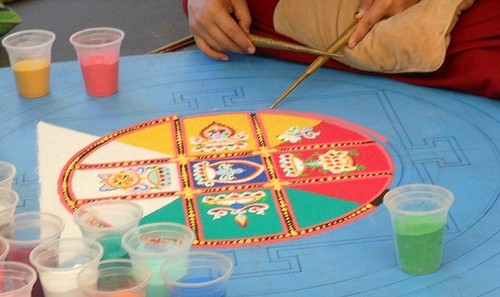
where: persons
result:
[183,0,500,102]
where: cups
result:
[2,29,55,99]
[383,184,454,275]
[0,186,19,223]
[0,160,16,189]
[72,200,143,259]
[78,258,152,297]
[68,27,124,96]
[0,260,37,297]
[158,252,234,297]
[28,237,103,297]
[0,236,10,261]
[0,212,65,297]
[120,224,194,297]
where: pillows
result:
[273,0,475,74]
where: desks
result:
[0,52,500,297]
